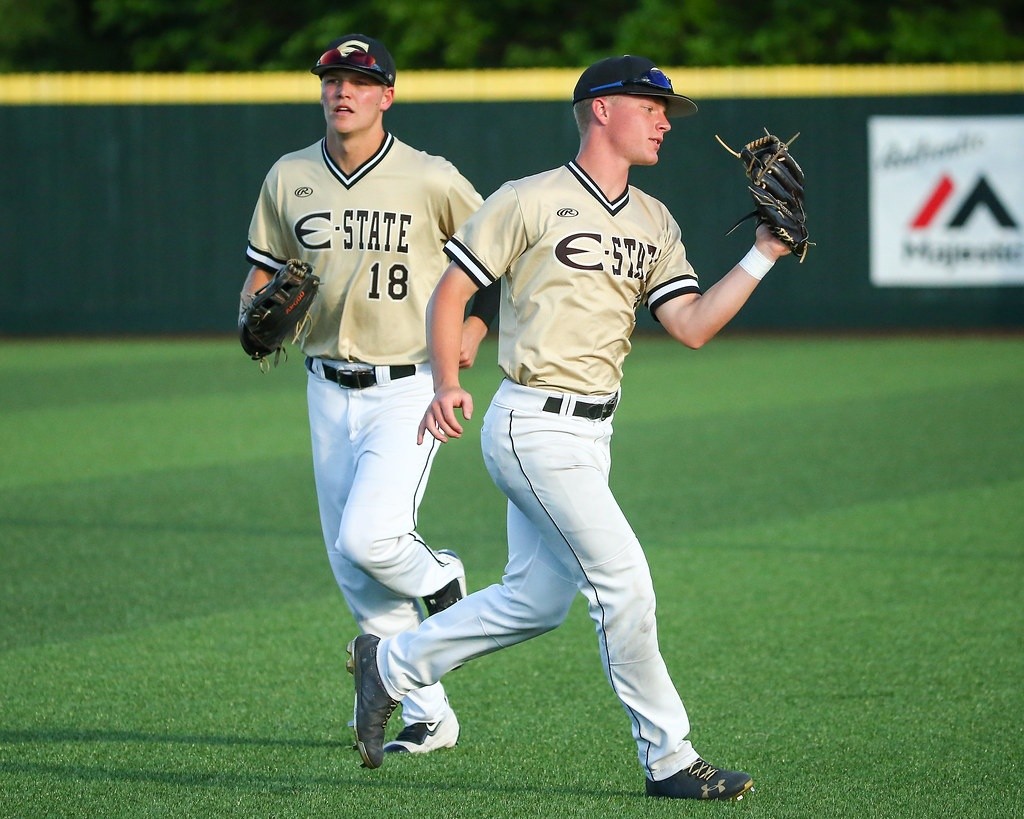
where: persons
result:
[347,56,810,800]
[237,33,501,753]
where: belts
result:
[542,392,618,421]
[304,355,415,389]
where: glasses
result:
[319,48,395,84]
[588,65,673,93]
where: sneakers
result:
[346,633,397,768]
[647,759,752,800]
[423,549,467,671]
[382,712,460,754]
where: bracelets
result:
[739,245,775,280]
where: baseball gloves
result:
[240,258,321,357]
[739,136,810,256]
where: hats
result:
[573,57,698,119]
[311,34,396,86]
